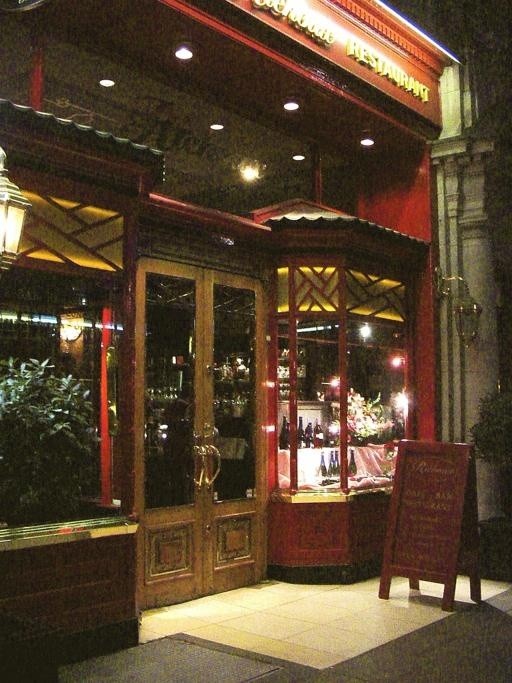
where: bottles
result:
[318,448,358,486]
[280,415,339,450]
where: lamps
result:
[434,266,480,347]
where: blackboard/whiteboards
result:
[384,438,471,585]
